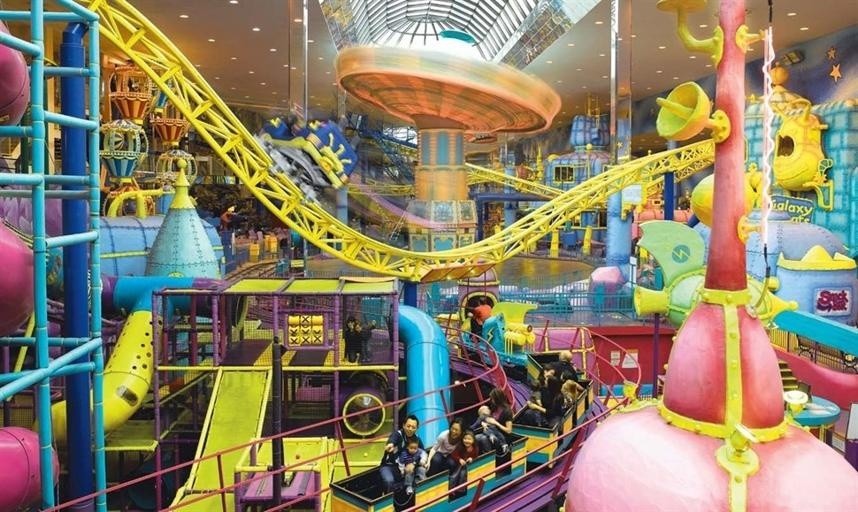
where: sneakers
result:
[405,485,414,495]
